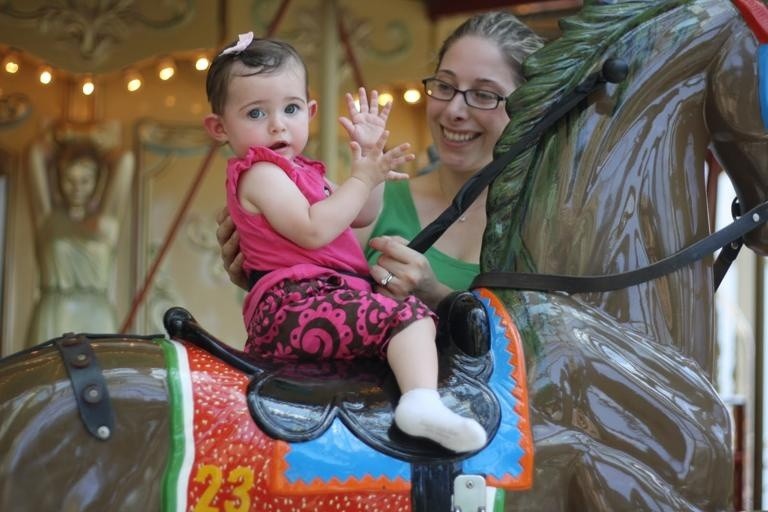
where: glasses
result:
[422,76,508,110]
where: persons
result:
[22,138,137,350]
